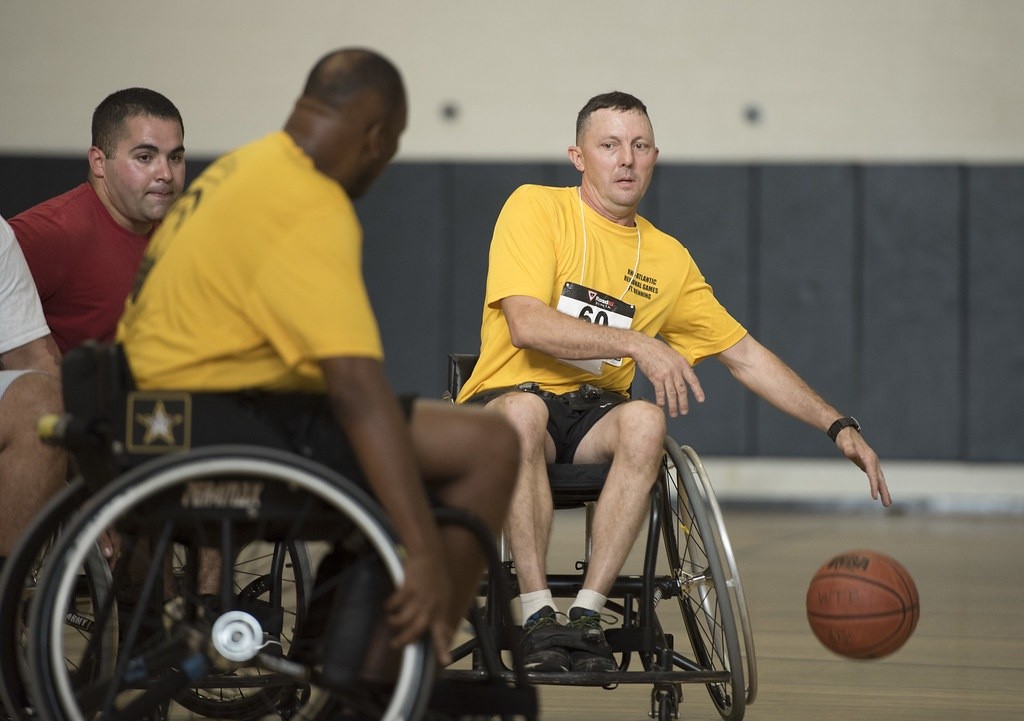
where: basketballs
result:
[802,547,925,664]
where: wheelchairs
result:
[440,350,762,721]
[0,340,542,721]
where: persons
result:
[8,88,222,618]
[0,213,71,578]
[453,90,892,675]
[114,49,522,667]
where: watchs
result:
[827,416,861,443]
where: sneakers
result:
[524,606,617,672]
